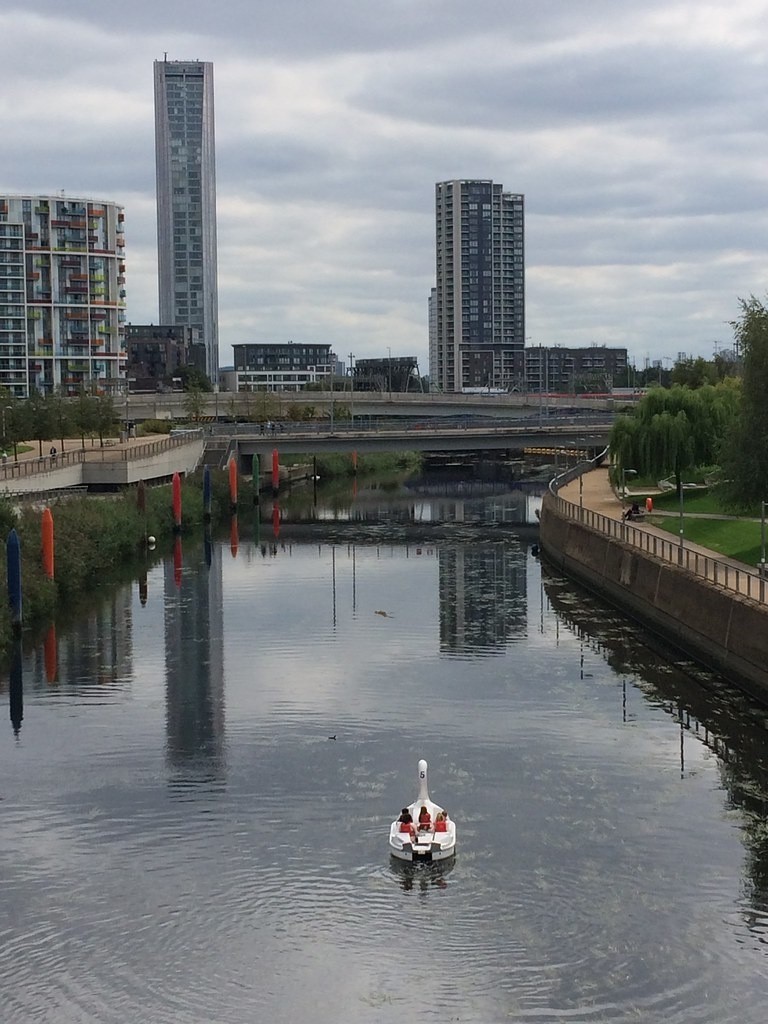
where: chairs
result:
[393,821,451,844]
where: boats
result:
[387,759,457,863]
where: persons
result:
[417,806,431,831]
[50,445,56,462]
[259,420,276,436]
[396,807,419,843]
[432,810,448,832]
[624,501,639,521]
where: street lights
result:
[214,385,220,423]
[622,468,637,509]
[125,397,130,442]
[347,352,355,431]
[680,481,697,551]
[579,458,592,509]
[2,405,12,466]
[386,347,392,398]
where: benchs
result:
[631,513,645,522]
[756,563,768,578]
